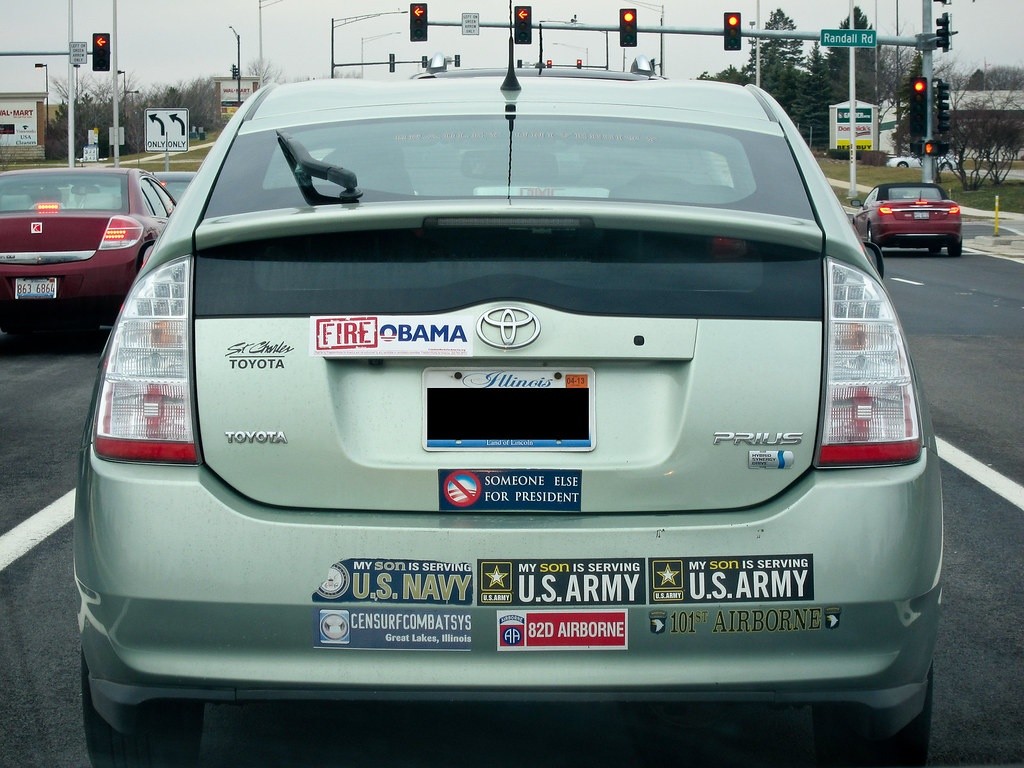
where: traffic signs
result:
[144,107,189,153]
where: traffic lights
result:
[909,77,929,137]
[723,12,741,51]
[909,142,924,156]
[409,3,428,42]
[935,12,949,53]
[937,80,951,132]
[939,141,949,155]
[925,140,940,155]
[515,6,532,44]
[92,33,110,72]
[620,8,637,47]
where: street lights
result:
[125,90,139,106]
[117,70,126,96]
[73,63,80,101]
[229,26,241,107]
[35,63,50,140]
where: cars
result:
[850,182,963,257]
[0,166,193,335]
[69,78,945,768]
